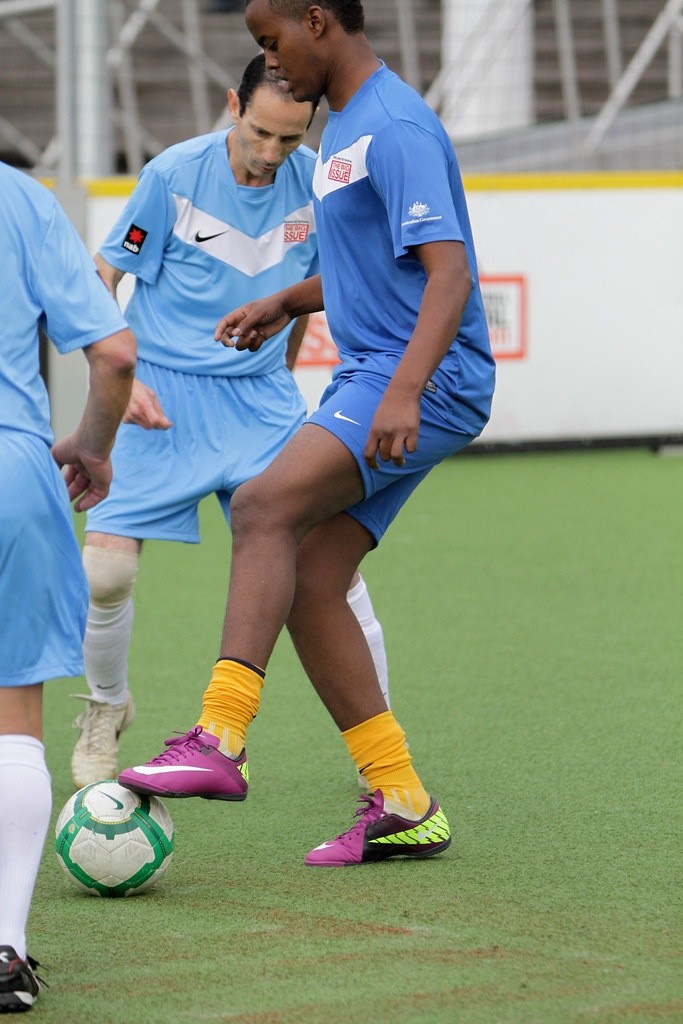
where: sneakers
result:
[0,945,50,1014]
[67,689,135,789]
[304,790,451,867]
[118,726,250,801]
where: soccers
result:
[54,778,178,892]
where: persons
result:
[1,158,137,1013]
[72,55,396,789]
[117,0,496,867]
